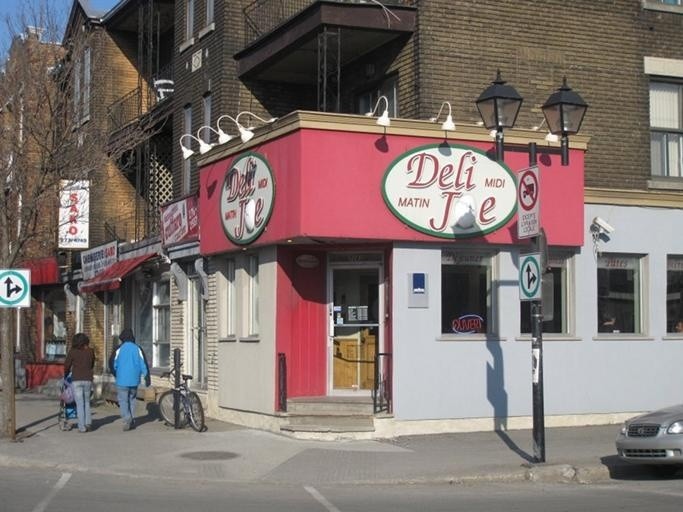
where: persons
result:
[674,319,683,332]
[63,333,95,434]
[109,328,151,431]
[599,310,620,332]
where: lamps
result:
[177,111,279,161]
[364,95,560,143]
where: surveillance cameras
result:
[593,217,614,236]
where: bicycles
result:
[154,365,205,434]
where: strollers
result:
[55,378,78,431]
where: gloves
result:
[144,375,150,386]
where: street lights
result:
[477,68,589,465]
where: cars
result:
[615,404,682,472]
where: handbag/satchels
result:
[60,380,74,404]
